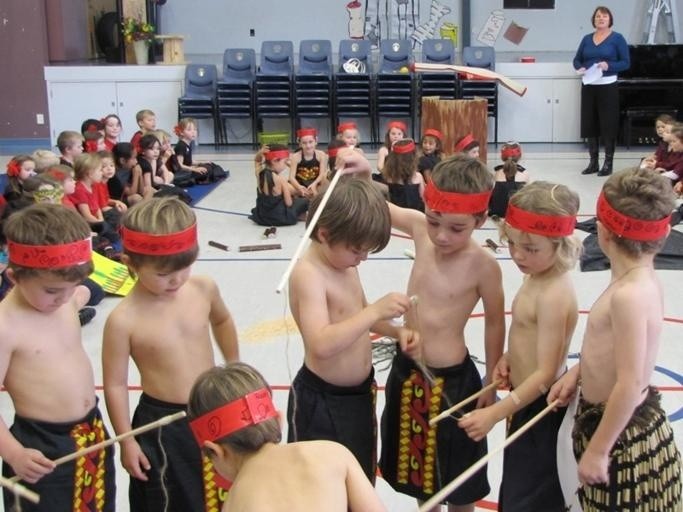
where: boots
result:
[582,137,599,174]
[598,141,615,176]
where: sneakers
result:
[79,308,95,324]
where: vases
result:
[133,41,150,65]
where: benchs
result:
[621,106,678,151]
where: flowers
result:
[120,16,154,41]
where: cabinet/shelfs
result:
[494,61,580,143]
[43,63,187,155]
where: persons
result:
[188,364,390,512]
[545,165,681,512]
[571,7,630,176]
[1,203,114,510]
[0,108,228,327]
[289,180,424,485]
[100,198,240,511]
[638,113,683,209]
[456,179,581,512]
[249,120,531,225]
[335,146,503,512]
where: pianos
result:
[618,44,683,146]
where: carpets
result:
[0,171,227,265]
